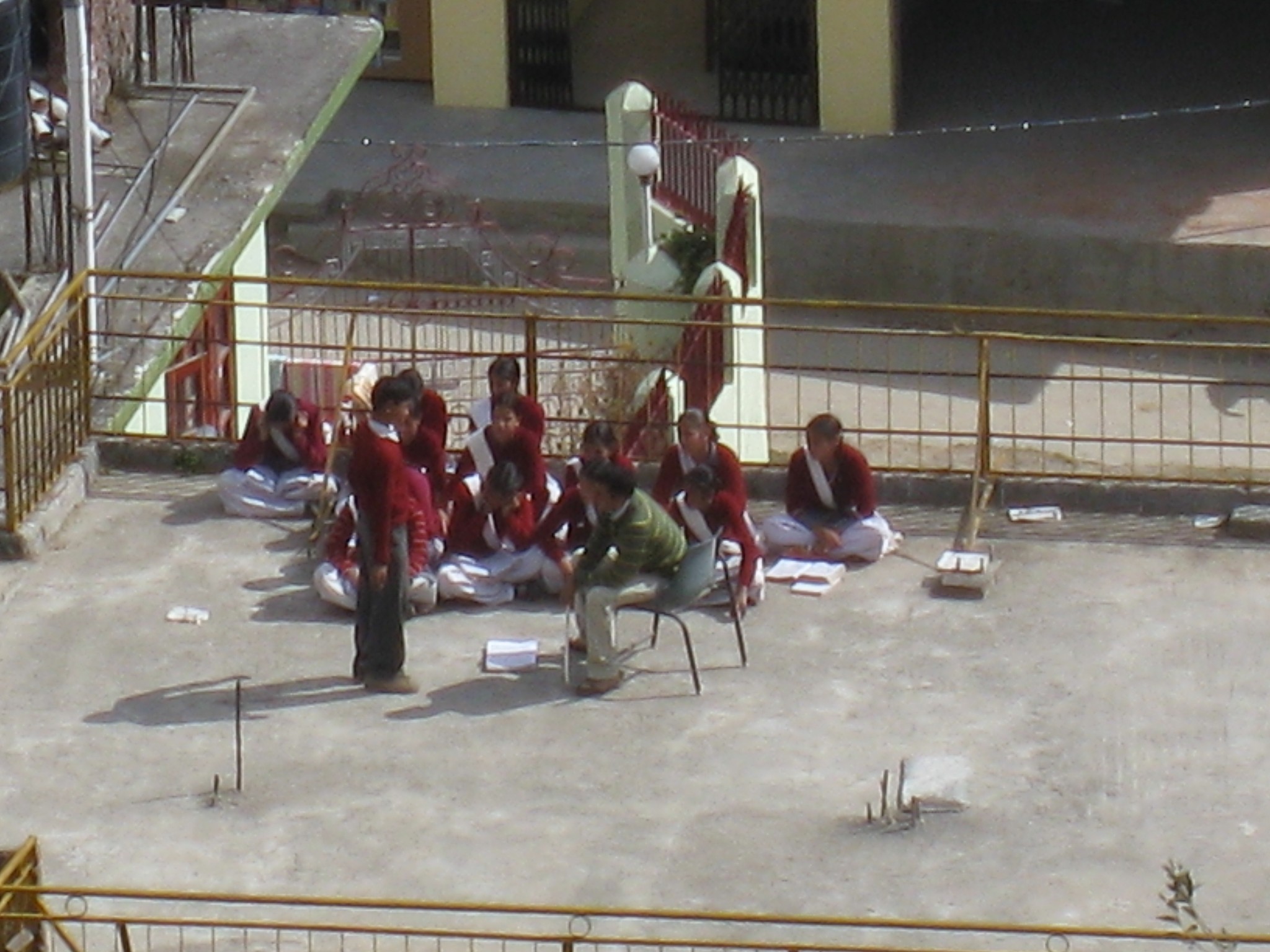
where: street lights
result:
[626,143,661,250]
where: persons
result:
[312,354,572,615]
[763,412,894,563]
[534,422,637,593]
[566,459,688,696]
[654,408,766,606]
[349,376,422,693]
[667,464,758,615]
[221,390,330,516]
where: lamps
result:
[625,144,662,249]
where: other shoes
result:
[780,543,825,561]
[576,677,621,697]
[569,636,588,653]
[354,669,420,694]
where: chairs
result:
[563,517,750,696]
